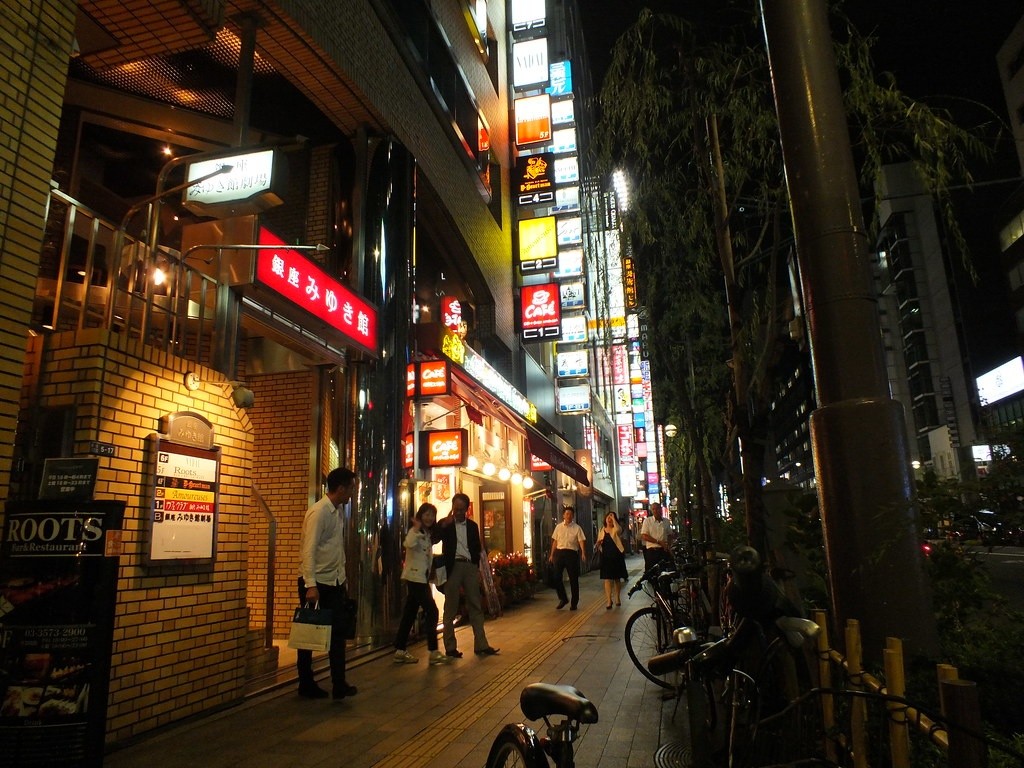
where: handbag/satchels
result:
[287,600,332,652]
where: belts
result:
[455,558,469,562]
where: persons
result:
[392,503,453,666]
[642,502,679,619]
[286,467,358,697]
[442,494,501,658]
[549,506,586,611]
[592,511,632,609]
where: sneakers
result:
[393,649,420,663]
[429,650,453,664]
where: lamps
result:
[183,373,256,409]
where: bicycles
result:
[674,537,717,641]
[486,653,760,768]
[625,558,719,730]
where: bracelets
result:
[657,539,658,543]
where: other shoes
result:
[299,688,328,698]
[570,606,577,610]
[474,646,500,656]
[333,686,357,699]
[446,650,463,656]
[556,599,568,609]
[616,602,621,606]
[606,601,613,609]
